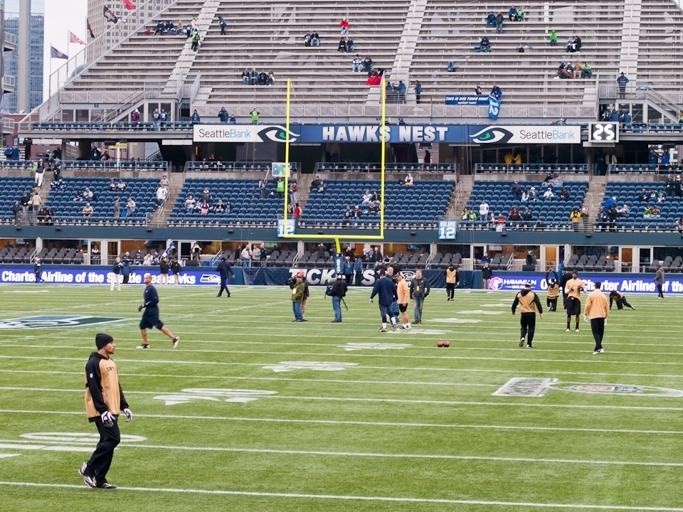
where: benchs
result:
[51,1,683,127]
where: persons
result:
[480,37,490,52]
[75,332,134,492]
[131,107,261,127]
[218,16,227,35]
[153,16,201,51]
[548,30,593,79]
[474,86,502,100]
[337,18,384,80]
[304,31,320,47]
[486,5,524,34]
[33,257,44,284]
[77,243,664,356]
[603,72,631,128]
[385,80,422,103]
[241,67,275,85]
[2,145,675,234]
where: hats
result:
[96,333,114,349]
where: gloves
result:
[100,411,115,428]
[137,304,144,312]
[124,408,133,421]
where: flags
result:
[50,46,69,59]
[70,32,87,45]
[124,1,136,10]
[87,20,96,38]
[103,6,118,24]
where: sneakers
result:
[171,336,180,349]
[519,338,525,347]
[136,343,151,349]
[592,349,604,354]
[79,462,116,488]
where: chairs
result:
[1,154,683,273]
